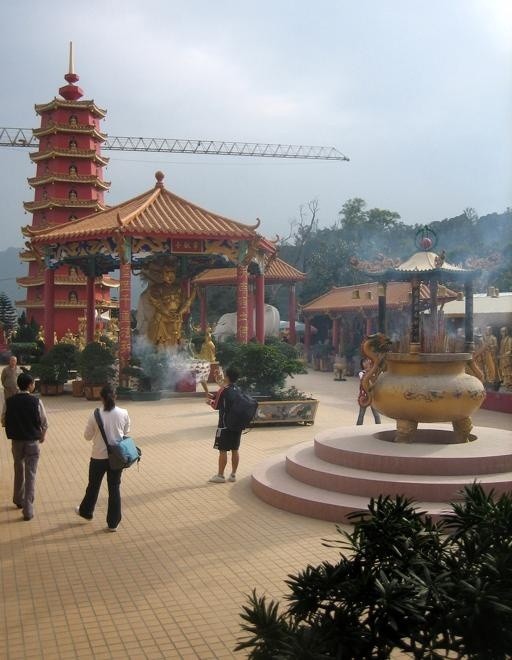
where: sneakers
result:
[209,474,236,483]
[13,499,33,520]
[75,506,93,520]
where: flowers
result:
[270,386,313,400]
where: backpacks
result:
[224,389,258,431]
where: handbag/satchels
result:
[107,437,141,470]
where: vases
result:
[249,395,320,426]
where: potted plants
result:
[10,340,164,400]
[308,343,335,370]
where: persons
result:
[205,367,242,482]
[356,357,381,426]
[2,374,48,520]
[147,264,190,355]
[2,356,23,430]
[457,326,511,389]
[76,385,131,532]
